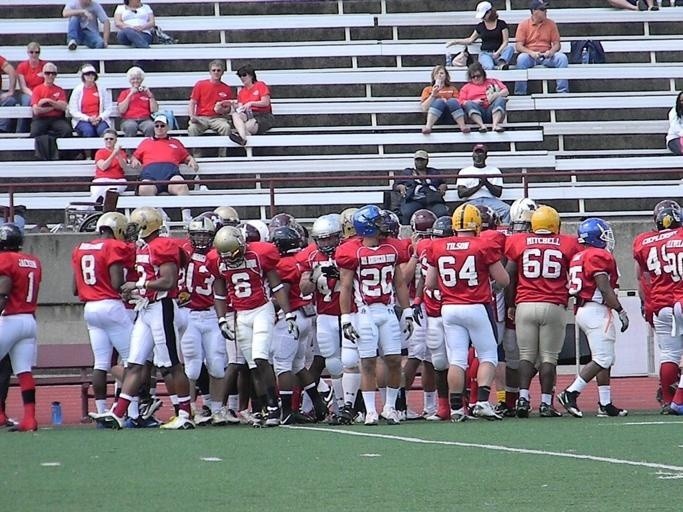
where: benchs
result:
[0,1,682,231]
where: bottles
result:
[581,48,587,64]
[192,174,200,191]
[656,0,662,8]
[669,0,674,7]
[445,49,454,66]
[164,110,174,130]
[50,400,62,428]
[432,77,440,88]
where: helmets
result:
[353,204,393,236]
[432,216,453,237]
[311,214,342,252]
[0,225,23,251]
[190,205,307,267]
[475,205,495,229]
[531,205,559,234]
[508,199,536,222]
[578,217,615,253]
[452,203,481,234]
[342,207,359,236]
[98,211,129,240]
[654,200,683,230]
[383,210,400,235]
[128,207,163,239]
[331,215,345,224]
[410,209,437,235]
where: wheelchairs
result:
[49,187,119,233]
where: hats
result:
[155,116,168,124]
[528,0,548,9]
[475,1,491,18]
[414,150,428,159]
[80,63,96,74]
[473,144,487,152]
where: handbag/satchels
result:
[569,40,605,64]
[451,42,469,66]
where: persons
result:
[114,0,155,70]
[230,65,275,155]
[188,60,232,157]
[118,67,158,137]
[0,222,42,432]
[419,63,470,134]
[633,198,682,416]
[390,150,450,224]
[457,60,510,133]
[30,63,72,138]
[62,0,110,49]
[69,66,112,160]
[131,116,199,195]
[513,0,570,96]
[606,0,682,12]
[663,91,682,155]
[74,197,631,428]
[441,0,514,71]
[454,142,512,227]
[1,56,17,106]
[17,43,46,107]
[90,129,129,195]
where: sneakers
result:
[659,402,683,415]
[88,386,581,429]
[0,416,38,432]
[230,132,247,145]
[598,403,628,417]
[422,125,504,134]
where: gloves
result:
[400,304,423,340]
[129,295,149,311]
[218,317,235,340]
[285,313,299,339]
[619,310,628,332]
[343,323,360,344]
[312,266,340,280]
